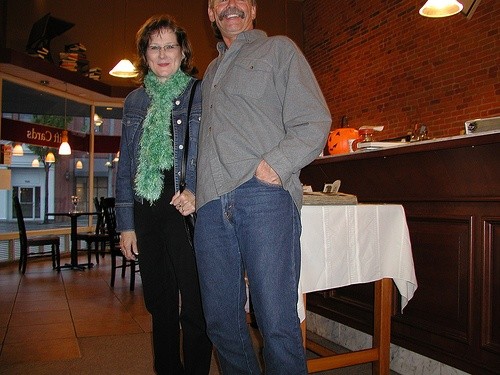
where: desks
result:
[244,203,417,374]
[45,210,106,272]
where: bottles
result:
[358,129,374,142]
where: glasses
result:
[147,44,180,53]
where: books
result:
[356,141,407,147]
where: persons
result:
[194,0,332,375]
[115,15,212,375]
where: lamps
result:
[45,149,56,162]
[33,154,41,167]
[11,143,25,157]
[105,152,121,167]
[76,161,83,169]
[419,0,463,18]
[94,114,104,127]
[59,97,71,155]
[109,0,138,79]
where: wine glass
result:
[71,196,79,213]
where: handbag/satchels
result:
[181,212,197,251]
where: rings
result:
[182,208,185,212]
[178,203,182,208]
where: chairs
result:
[77,196,139,291]
[10,186,63,274]
